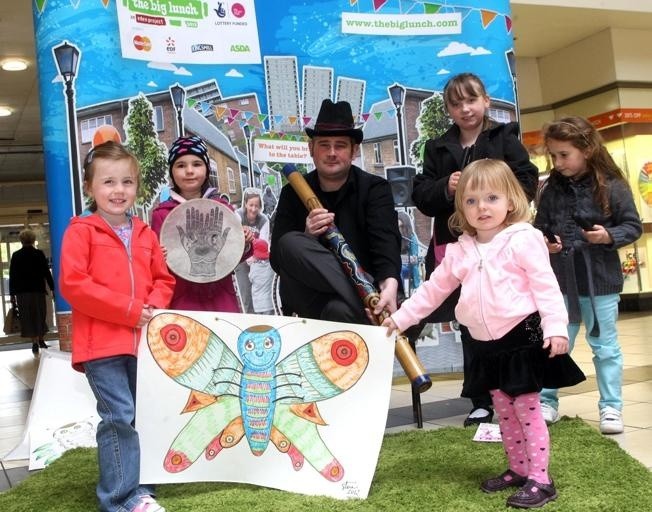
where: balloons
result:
[93,125,120,148]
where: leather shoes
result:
[507,479,557,508]
[481,469,528,493]
[39,342,47,348]
[33,344,38,352]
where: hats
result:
[305,100,363,144]
[167,135,209,166]
[252,239,270,259]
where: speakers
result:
[386,166,417,207]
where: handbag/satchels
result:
[434,241,458,267]
[4,306,22,334]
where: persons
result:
[151,136,253,312]
[380,158,569,508]
[413,73,539,428]
[60,141,174,511]
[10,229,55,354]
[534,116,643,436]
[269,136,402,329]
[232,188,270,311]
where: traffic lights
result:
[388,167,415,207]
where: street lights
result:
[51,37,83,218]
[241,125,256,186]
[504,47,522,141]
[166,81,187,138]
[388,82,408,164]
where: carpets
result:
[0,416,652,511]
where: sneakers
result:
[541,402,560,423]
[599,406,624,433]
[464,406,493,428]
[131,495,165,512]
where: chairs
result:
[271,266,423,428]
